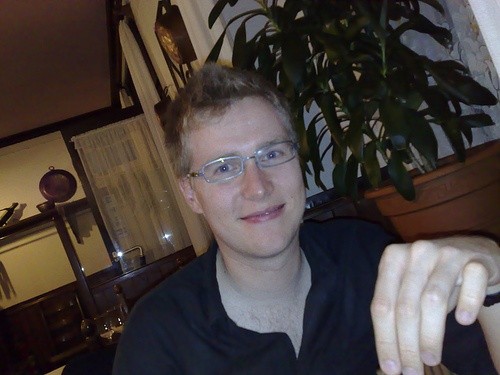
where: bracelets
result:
[483,290,500,307]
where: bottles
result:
[0,202,18,227]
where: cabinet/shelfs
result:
[0,196,196,375]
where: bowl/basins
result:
[37,201,53,212]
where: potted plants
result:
[204,0,500,244]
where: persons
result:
[112,60,500,375]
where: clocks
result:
[153,7,199,64]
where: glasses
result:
[185,139,303,184]
